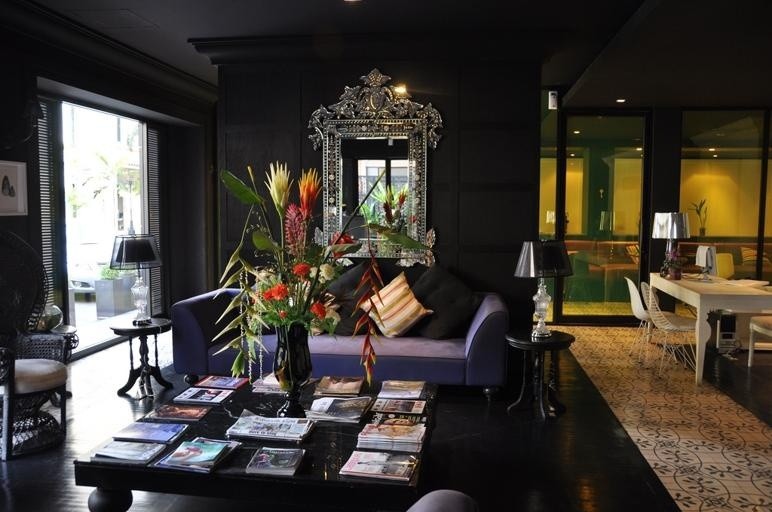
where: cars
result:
[65,239,121,298]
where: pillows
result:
[718,248,741,265]
[315,260,476,340]
[739,246,771,266]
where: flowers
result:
[357,179,409,234]
[215,161,362,386]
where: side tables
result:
[110,318,172,400]
[504,330,575,422]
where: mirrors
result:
[308,68,445,269]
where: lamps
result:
[111,234,165,326]
[600,188,604,198]
[513,239,575,338]
[651,212,691,280]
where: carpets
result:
[546,303,772,512]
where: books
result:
[340,380,428,481]
[227,373,376,476]
[95,375,250,474]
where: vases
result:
[273,322,313,418]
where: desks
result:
[648,271,772,384]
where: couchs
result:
[565,240,640,273]
[168,261,509,404]
[675,240,772,281]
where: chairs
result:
[0,222,67,461]
[623,277,714,387]
[747,315,772,368]
